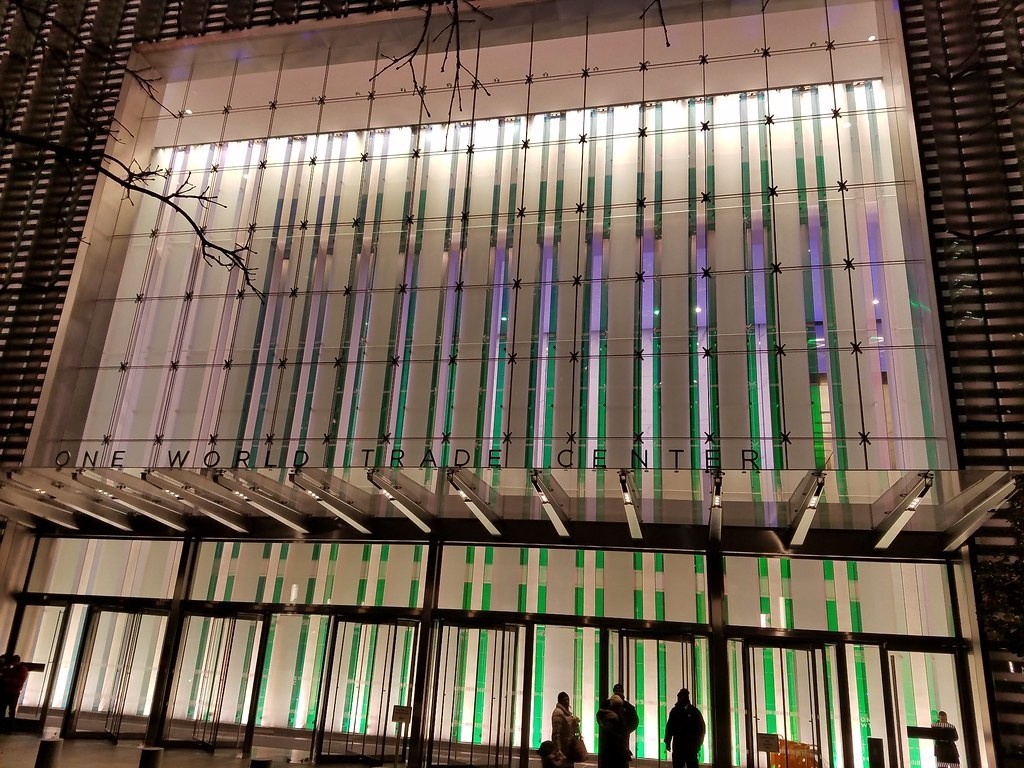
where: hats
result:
[610,695,623,706]
[539,741,554,757]
[558,692,568,703]
[613,684,624,693]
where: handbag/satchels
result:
[571,728,590,763]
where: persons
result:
[596,683,639,768]
[932,711,961,768]
[552,691,579,768]
[664,688,706,768]
[537,740,560,768]
[0,654,29,735]
